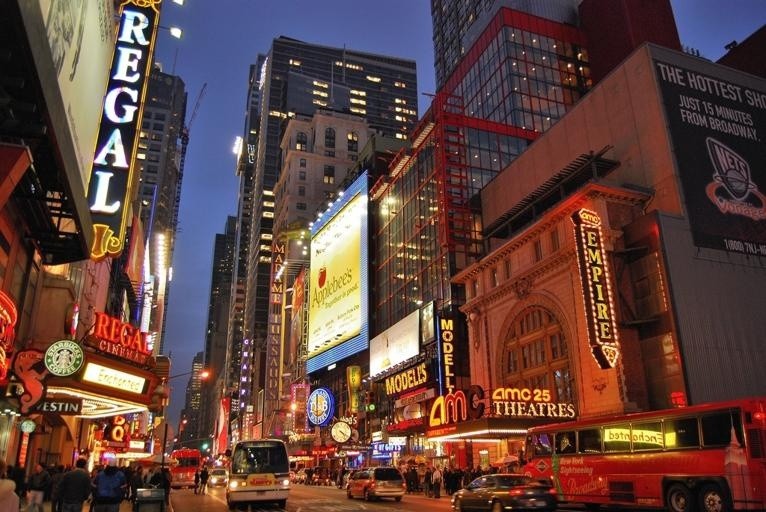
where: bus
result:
[227,438,291,509]
[168,445,203,489]
[521,395,766,511]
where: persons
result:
[0,452,211,511]
[300,463,489,498]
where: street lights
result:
[161,369,209,470]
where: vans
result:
[347,466,406,502]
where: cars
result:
[206,468,229,488]
[450,472,561,511]
[289,466,331,486]
